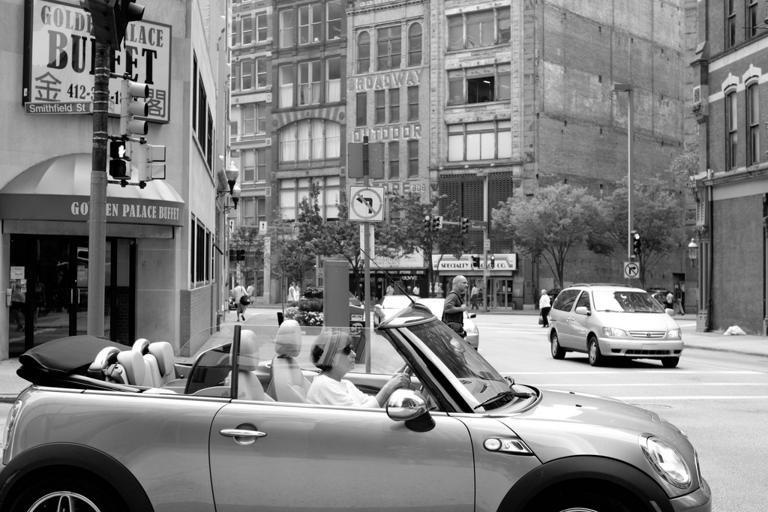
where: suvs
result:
[0,247,713,510]
[547,282,687,368]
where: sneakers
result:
[237,315,245,321]
[543,324,549,327]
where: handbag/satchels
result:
[240,296,250,305]
[446,321,465,337]
[539,318,548,324]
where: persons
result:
[674,283,685,315]
[304,329,411,411]
[538,288,552,328]
[385,283,395,295]
[665,291,674,308]
[286,282,296,307]
[295,281,301,306]
[440,275,468,338]
[231,281,250,322]
[469,284,480,309]
[412,283,421,296]
[247,280,255,304]
[437,283,445,297]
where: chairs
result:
[106,319,312,405]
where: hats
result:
[311,331,349,372]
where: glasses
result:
[343,345,354,355]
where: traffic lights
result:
[108,140,132,180]
[630,230,642,255]
[119,79,150,137]
